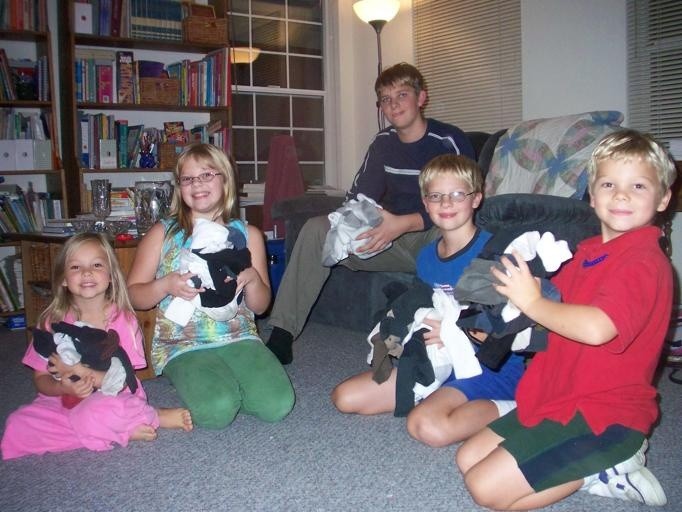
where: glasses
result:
[424,190,477,203]
[175,171,222,187]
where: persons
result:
[331,154,527,448]
[125,142,297,430]
[456,130,677,511]
[266,62,476,365]
[1,233,194,460]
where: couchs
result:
[272,129,602,355]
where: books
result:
[1,0,230,329]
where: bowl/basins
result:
[71,220,132,239]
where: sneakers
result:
[583,439,669,508]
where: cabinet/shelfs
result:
[1,230,156,383]
[0,0,70,316]
[60,0,232,213]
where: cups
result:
[135,181,173,236]
[90,176,111,220]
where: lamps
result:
[228,46,258,127]
[351,0,402,133]
